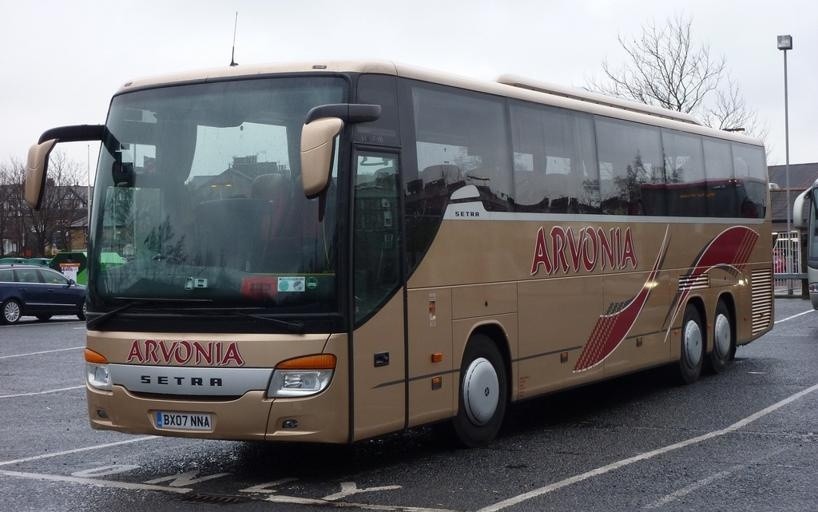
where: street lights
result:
[774,36,794,296]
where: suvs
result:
[0,263,89,324]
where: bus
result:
[791,177,817,311]
[23,11,776,452]
